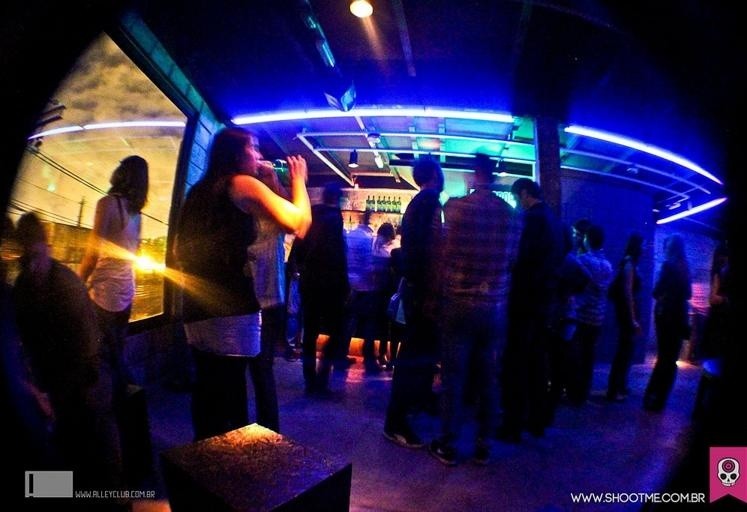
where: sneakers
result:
[381,422,488,466]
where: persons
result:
[243,160,291,435]
[75,156,147,364]
[171,125,313,438]
[606,233,644,402]
[695,243,728,407]
[283,153,616,469]
[12,214,124,474]
[641,235,693,412]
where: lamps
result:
[347,150,360,169]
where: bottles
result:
[365,195,402,213]
[260,159,290,176]
